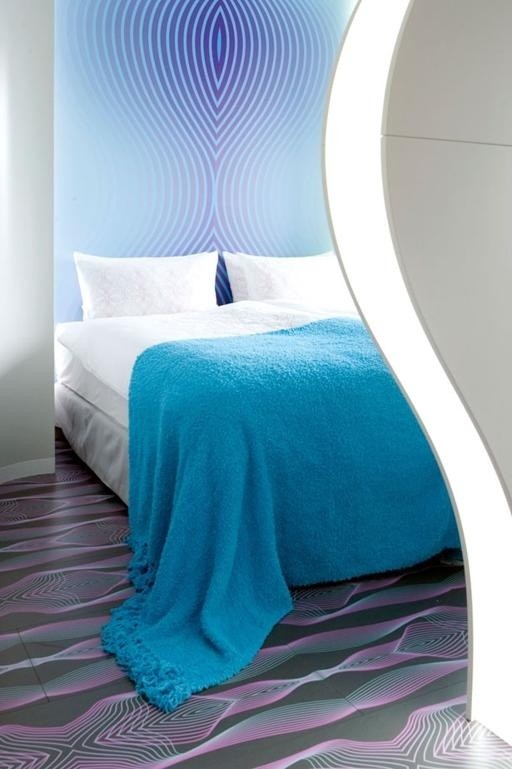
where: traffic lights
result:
[73,250,220,320]
[222,250,344,302]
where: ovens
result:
[54,300,465,711]
